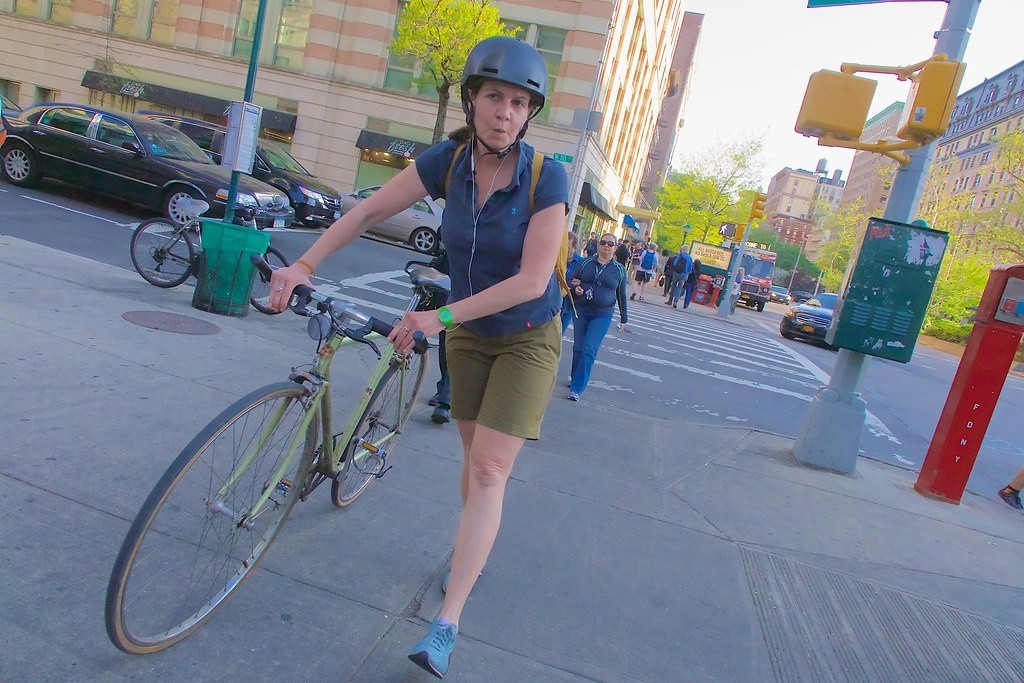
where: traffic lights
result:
[718,220,736,237]
[736,225,746,240]
[750,191,768,219]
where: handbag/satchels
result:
[658,274,665,286]
[688,265,697,285]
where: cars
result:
[339,184,445,255]
[771,285,814,305]
[779,293,842,353]
[0,98,297,230]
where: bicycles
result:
[130,196,294,314]
[104,249,453,656]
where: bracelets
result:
[297,260,313,273]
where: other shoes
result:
[630,291,637,300]
[431,403,450,424]
[428,392,440,405]
[665,301,672,304]
[673,304,677,308]
[639,296,643,300]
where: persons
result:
[429,328,450,424]
[664,245,692,308]
[654,249,669,287]
[567,234,628,401]
[562,233,583,334]
[683,260,701,308]
[627,241,645,284]
[614,239,629,267]
[268,38,570,677]
[730,267,745,315]
[630,243,658,300]
[585,232,598,257]
[996,465,1024,513]
[662,252,679,296]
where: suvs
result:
[131,108,343,229]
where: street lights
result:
[679,223,691,253]
[786,217,806,292]
[808,212,829,297]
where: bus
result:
[722,246,778,312]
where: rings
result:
[275,288,283,291]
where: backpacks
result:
[641,250,655,270]
[671,254,686,277]
[444,141,569,296]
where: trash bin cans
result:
[192,218,271,319]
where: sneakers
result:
[408,618,457,679]
[442,570,451,592]
[997,490,1024,514]
[567,376,572,385]
[567,391,579,401]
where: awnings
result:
[81,69,296,135]
[624,214,640,233]
[578,182,616,222]
[354,129,430,158]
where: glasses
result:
[599,240,614,247]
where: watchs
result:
[438,307,452,327]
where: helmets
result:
[460,36,548,109]
